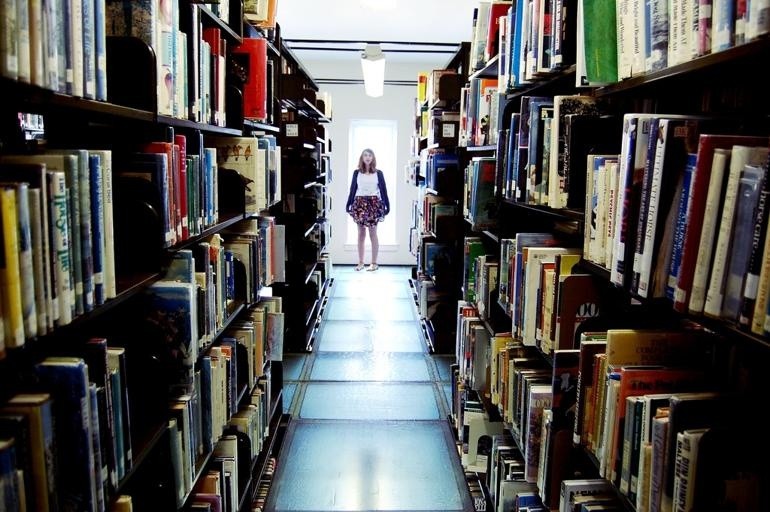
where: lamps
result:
[355,39,393,100]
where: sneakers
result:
[366,263,378,271]
[354,262,364,271]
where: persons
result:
[346,149,390,271]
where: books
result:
[1,1,331,247]
[447,229,770,512]
[411,0,770,338]
[1,145,332,511]
